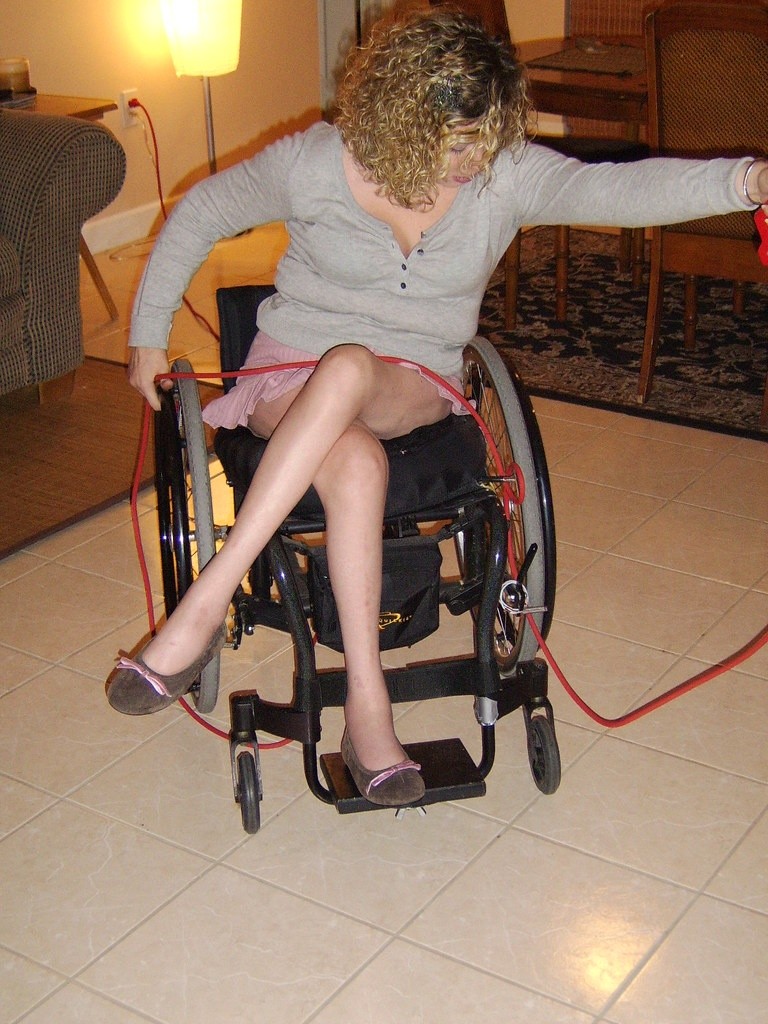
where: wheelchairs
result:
[151,280,565,835]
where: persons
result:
[106,1,768,806]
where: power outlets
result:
[120,88,138,127]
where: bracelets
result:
[743,158,766,205]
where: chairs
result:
[394,0,768,406]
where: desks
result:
[509,33,653,323]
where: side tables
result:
[1,93,120,321]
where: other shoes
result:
[340,725,426,809]
[106,621,228,716]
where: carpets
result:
[462,225,768,442]
[1,355,226,559]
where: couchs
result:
[0,108,128,406]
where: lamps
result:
[162,0,253,237]
